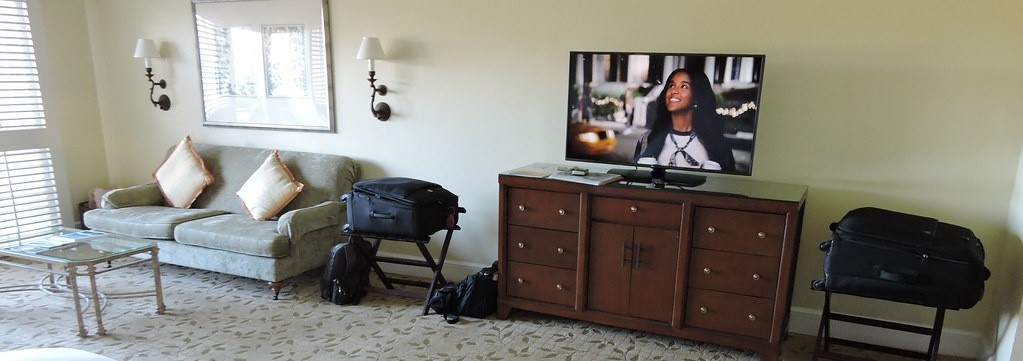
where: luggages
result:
[339,177,459,240]
[812,207,991,311]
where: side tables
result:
[806,283,960,361]
[337,205,467,315]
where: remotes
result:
[652,178,665,187]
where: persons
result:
[633,68,736,172]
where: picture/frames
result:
[190,0,336,133]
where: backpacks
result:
[320,235,373,306]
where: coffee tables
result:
[0,224,166,339]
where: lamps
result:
[355,36,392,122]
[133,39,171,112]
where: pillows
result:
[152,134,216,208]
[234,149,305,221]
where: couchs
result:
[82,143,362,301]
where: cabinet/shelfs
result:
[582,190,684,329]
[682,196,792,347]
[496,174,585,322]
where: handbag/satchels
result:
[427,260,499,324]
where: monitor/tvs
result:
[565,50,767,176]
